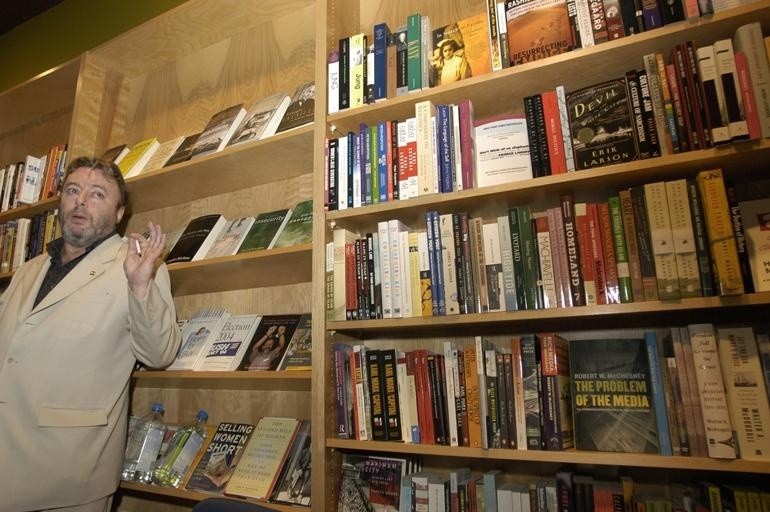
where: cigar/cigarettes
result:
[135,239,142,256]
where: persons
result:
[0,156,181,512]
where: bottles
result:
[120,403,167,490]
[150,411,209,490]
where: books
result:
[0,144,68,275]
[327,0,714,114]
[337,453,770,512]
[165,200,314,266]
[167,308,313,372]
[332,324,770,462]
[329,22,770,211]
[328,168,770,322]
[159,416,312,506]
[103,82,315,180]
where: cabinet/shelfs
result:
[321,1,770,512]
[1,54,82,289]
[70,0,317,510]
